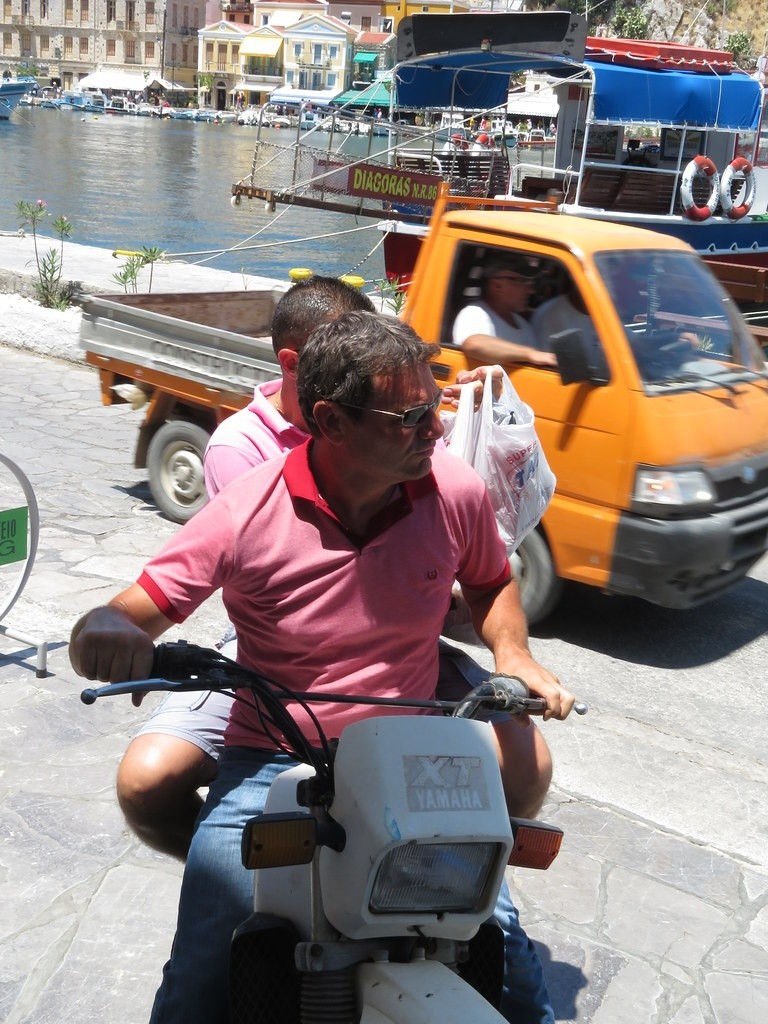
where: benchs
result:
[401,150,512,199]
[569,164,746,218]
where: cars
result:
[42,86,62,97]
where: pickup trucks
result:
[73,179,767,628]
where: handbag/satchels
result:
[443,365,556,559]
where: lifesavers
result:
[681,156,721,221]
[471,134,498,193]
[721,159,757,218]
[440,133,470,190]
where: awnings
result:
[353,53,378,63]
[332,83,397,107]
[234,81,280,92]
[80,70,155,93]
[238,35,284,59]
[270,86,343,106]
[424,91,560,117]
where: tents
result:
[149,78,198,100]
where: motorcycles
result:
[81,637,584,1024]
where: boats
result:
[1,74,38,120]
[20,93,398,138]
[434,112,548,148]
[232,0,768,339]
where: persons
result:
[236,93,245,111]
[372,108,383,119]
[301,98,312,113]
[469,115,487,133]
[58,273,585,1024]
[450,252,701,380]
[516,118,557,137]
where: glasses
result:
[339,388,444,427]
[487,274,535,285]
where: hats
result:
[480,252,547,280]
